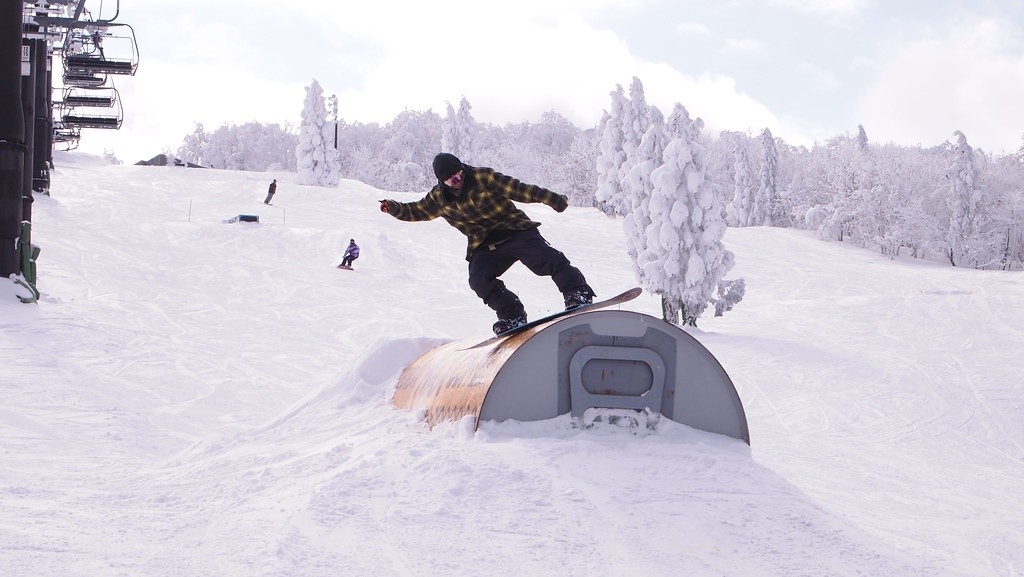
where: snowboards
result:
[452,287,642,352]
[336,266,354,271]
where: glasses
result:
[445,172,465,185]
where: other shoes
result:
[492,310,527,334]
[564,285,597,311]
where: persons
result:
[379,153,598,334]
[339,239,360,267]
[264,179,277,204]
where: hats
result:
[433,152,463,182]
